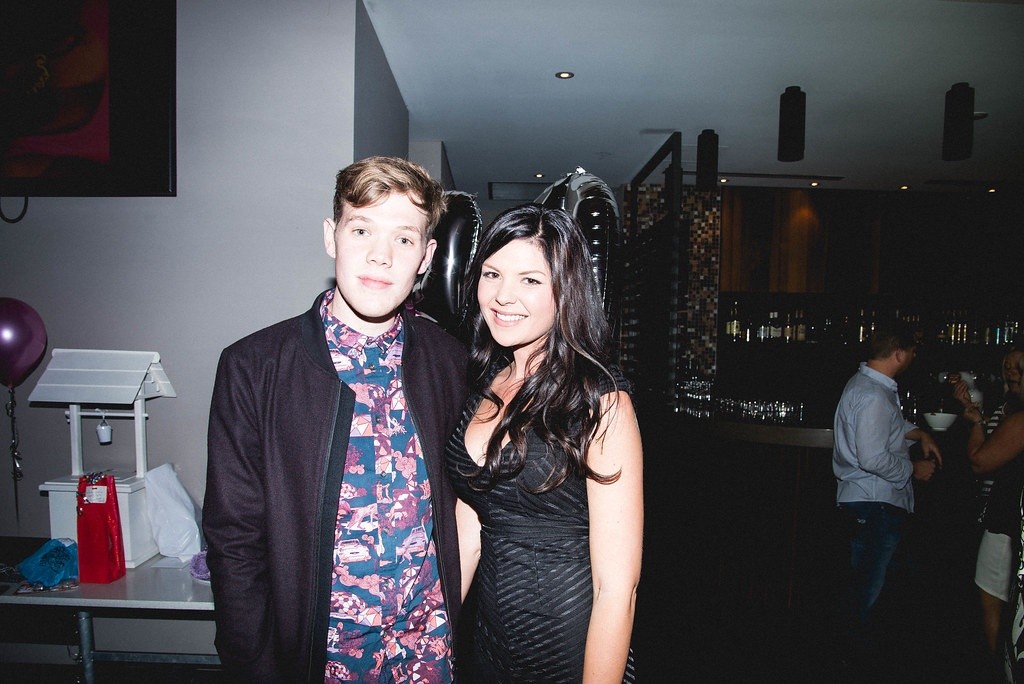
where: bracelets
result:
[971,420,987,425]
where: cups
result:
[715,398,805,421]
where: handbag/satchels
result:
[143,463,207,563]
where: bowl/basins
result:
[924,412,958,431]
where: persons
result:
[831,324,942,666]
[202,156,471,684]
[952,349,1024,674]
[445,204,643,684]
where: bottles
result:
[935,309,978,346]
[726,301,752,343]
[859,308,877,343]
[983,304,1024,346]
[823,313,832,343]
[783,309,818,343]
[840,316,850,347]
[895,310,925,344]
[756,312,780,344]
[900,390,920,427]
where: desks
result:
[0,535,221,684]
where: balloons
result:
[412,189,482,334]
[0,297,47,391]
[533,171,620,353]
